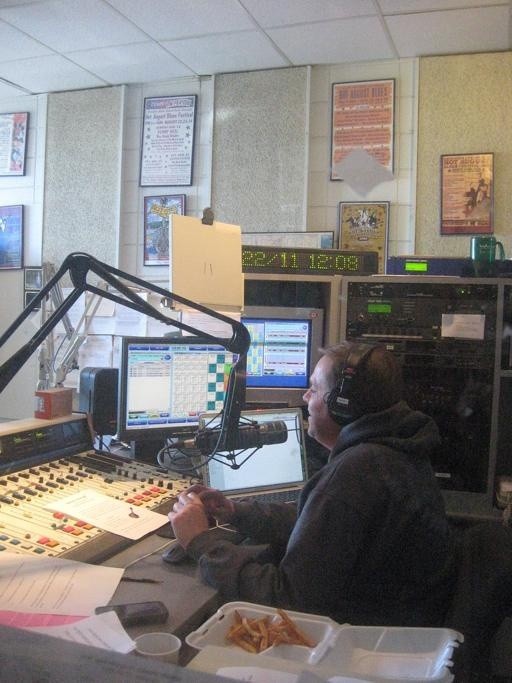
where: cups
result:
[133,631,182,665]
[470,235,505,278]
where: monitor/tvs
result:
[117,336,240,442]
[240,306,324,407]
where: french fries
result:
[228,609,316,654]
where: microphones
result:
[169,420,288,454]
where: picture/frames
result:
[138,94,197,186]
[242,231,333,249]
[144,194,186,266]
[24,266,43,291]
[338,202,390,275]
[440,152,495,234]
[330,77,396,182]
[0,205,24,270]
[24,291,41,312]
[0,111,30,177]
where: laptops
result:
[200,408,310,504]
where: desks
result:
[91,531,274,664]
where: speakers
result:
[79,367,118,435]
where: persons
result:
[169,337,450,629]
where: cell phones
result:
[96,601,169,627]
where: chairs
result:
[444,522,512,683]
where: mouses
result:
[163,543,188,564]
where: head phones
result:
[328,343,381,425]
